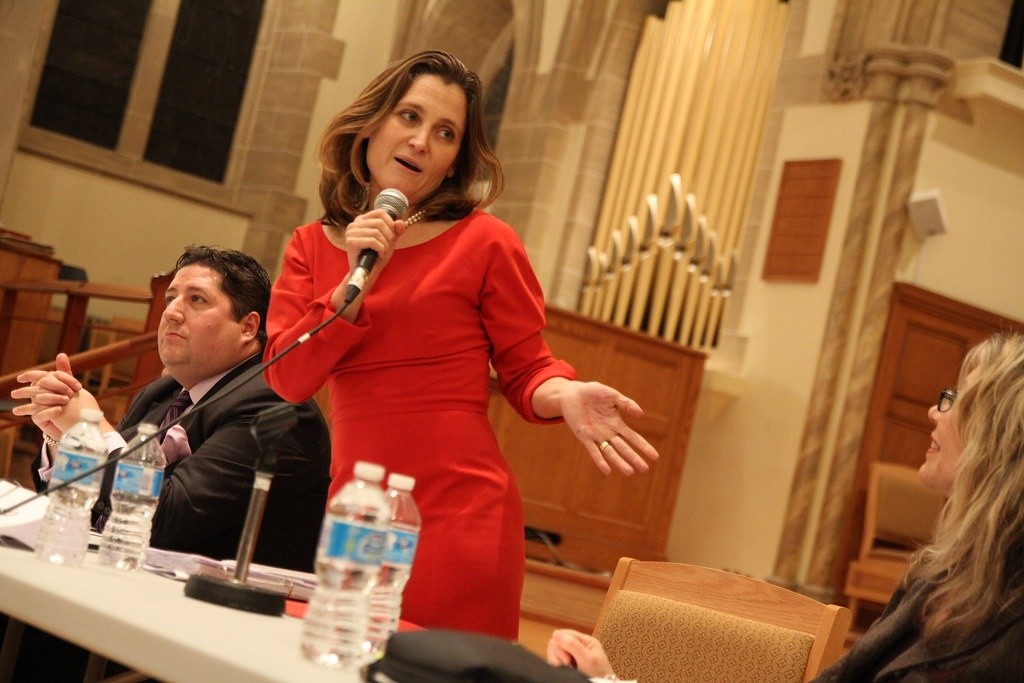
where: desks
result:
[0,479,403,683]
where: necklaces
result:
[404,208,426,227]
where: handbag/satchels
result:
[360,631,592,683]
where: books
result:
[88,531,101,546]
[144,538,319,603]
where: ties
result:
[158,389,193,446]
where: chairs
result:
[596,557,853,683]
[845,462,948,633]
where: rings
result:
[600,441,608,451]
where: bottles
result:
[32,407,109,568]
[97,422,167,575]
[299,461,390,669]
[363,472,423,664]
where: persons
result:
[545,332,1024,683]
[11,242,332,575]
[262,49,658,641]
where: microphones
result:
[345,189,409,302]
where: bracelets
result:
[43,432,59,448]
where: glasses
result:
[936,388,972,414]
